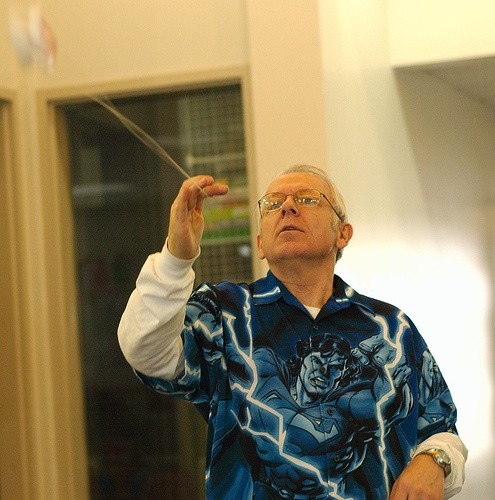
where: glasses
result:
[258,188,343,224]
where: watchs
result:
[414,448,451,478]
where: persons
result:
[118,165,468,500]
